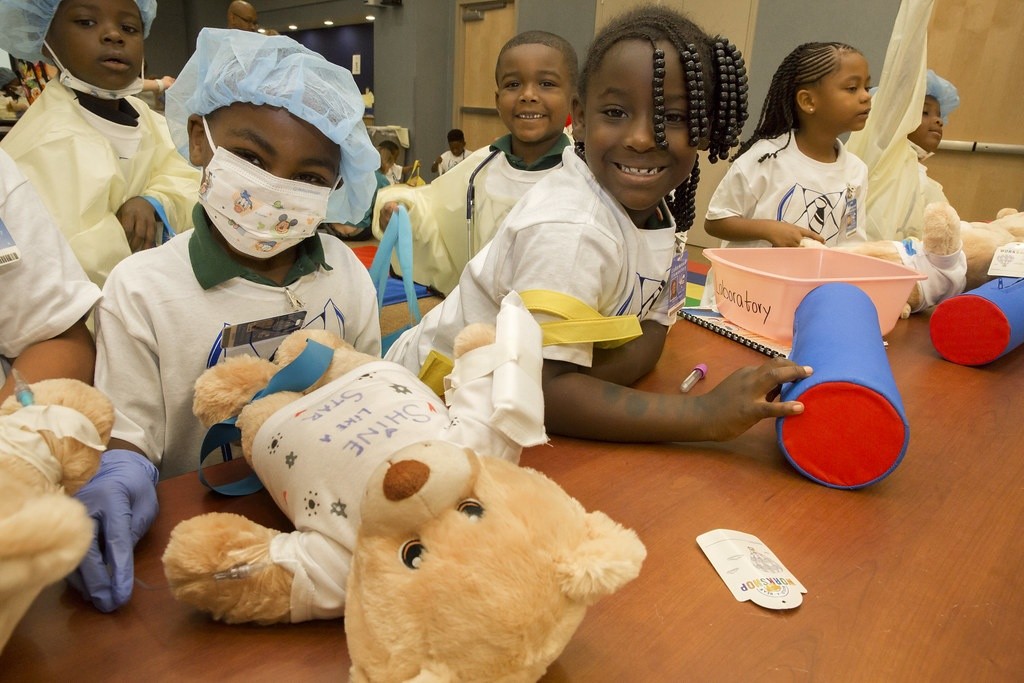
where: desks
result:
[0,313,1024,683]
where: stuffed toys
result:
[0,378,115,654]
[831,207,1024,319]
[161,323,647,683]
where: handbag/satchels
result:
[407,176,426,188]
[381,325,413,359]
[417,349,456,396]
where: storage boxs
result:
[702,247,926,339]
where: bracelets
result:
[156,79,164,91]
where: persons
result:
[700,42,872,306]
[0,67,19,100]
[0,0,382,611]
[372,29,580,299]
[140,76,176,92]
[844,0,959,241]
[326,130,473,242]
[383,8,815,444]
[227,0,281,36]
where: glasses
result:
[233,13,258,25]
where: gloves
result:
[64,449,160,613]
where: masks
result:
[197,115,342,259]
[43,39,144,100]
